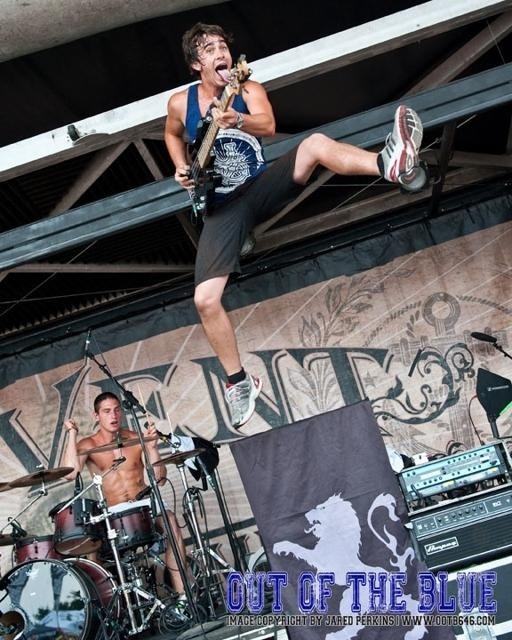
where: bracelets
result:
[235,111,245,130]
[66,425,81,436]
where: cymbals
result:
[9,467,74,487]
[0,483,13,492]
[151,449,206,466]
[76,436,162,454]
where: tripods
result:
[171,466,270,624]
[57,457,197,640]
[140,546,180,621]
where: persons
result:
[57,390,202,604]
[164,21,429,430]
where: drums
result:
[16,535,79,566]
[50,497,105,556]
[98,505,160,564]
[1,558,120,640]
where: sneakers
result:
[379,105,423,184]
[224,372,262,429]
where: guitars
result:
[187,54,252,219]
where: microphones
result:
[144,421,177,448]
[135,477,163,501]
[74,472,83,526]
[471,332,497,343]
[83,327,92,365]
[8,521,26,537]
